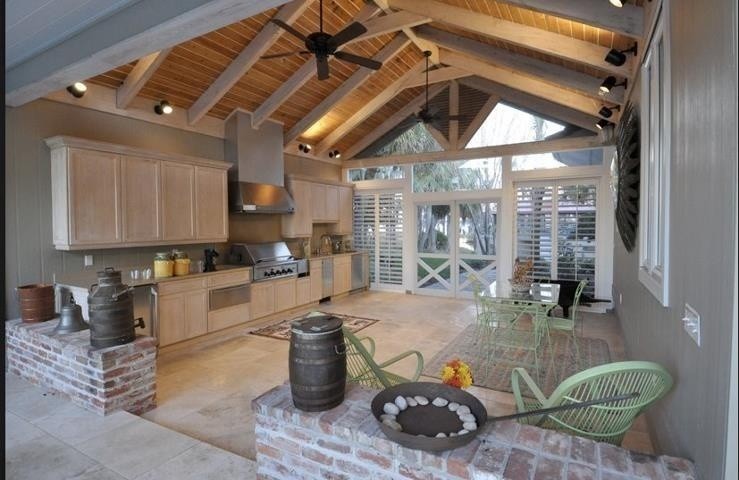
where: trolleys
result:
[260,1,384,82]
[397,51,467,128]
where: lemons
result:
[318,234,333,256]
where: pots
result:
[369,379,640,454]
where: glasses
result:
[299,143,311,154]
[154,100,173,115]
[599,75,629,94]
[609,0,642,9]
[329,149,341,159]
[597,104,622,118]
[603,41,639,67]
[596,119,615,131]
[65,82,87,99]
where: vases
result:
[440,359,475,391]
[512,257,532,279]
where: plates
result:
[42,134,234,252]
[312,182,340,225]
[210,268,252,332]
[309,251,370,306]
[327,186,353,236]
[297,275,311,307]
[281,173,313,238]
[252,277,296,320]
[157,275,209,348]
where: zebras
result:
[231,240,298,282]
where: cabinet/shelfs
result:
[172,252,191,276]
[335,241,344,249]
[154,253,172,278]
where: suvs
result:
[18,283,55,322]
[88,266,146,348]
[171,250,191,275]
[288,315,347,411]
[153,252,175,277]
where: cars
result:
[141,267,152,280]
[191,259,203,272]
[130,268,140,281]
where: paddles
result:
[249,309,380,341]
[421,323,612,399]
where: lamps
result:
[510,360,675,447]
[469,273,589,388]
[341,325,425,394]
